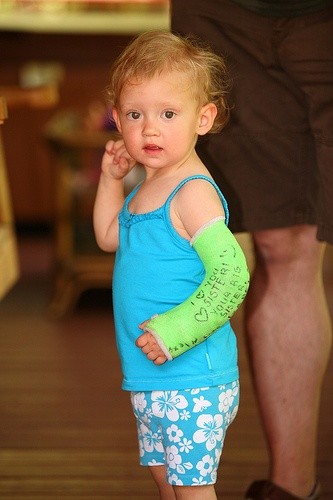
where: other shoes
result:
[243,477,324,500]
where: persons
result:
[94,31,250,499]
[171,0,333,500]
